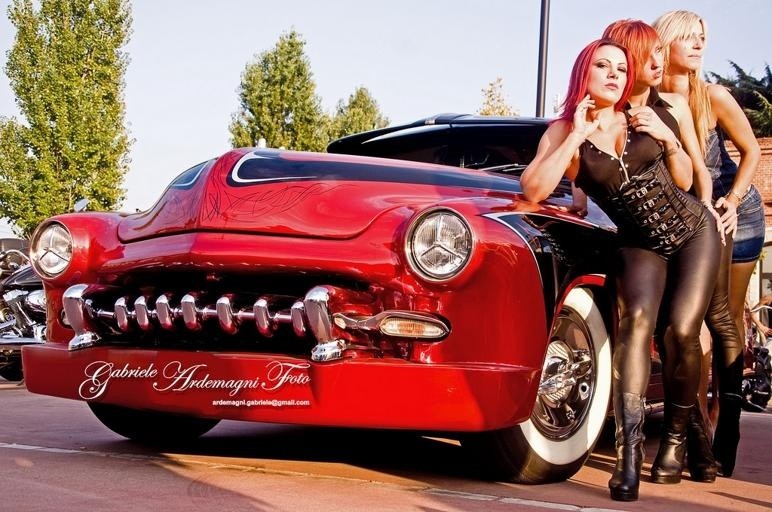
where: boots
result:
[608,392,646,502]
[651,399,695,484]
[687,398,717,482]
[711,393,743,477]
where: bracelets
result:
[699,200,715,211]
[727,190,744,203]
[662,146,683,157]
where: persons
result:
[520,41,726,500]
[750,292,772,364]
[651,10,765,476]
[568,18,741,483]
